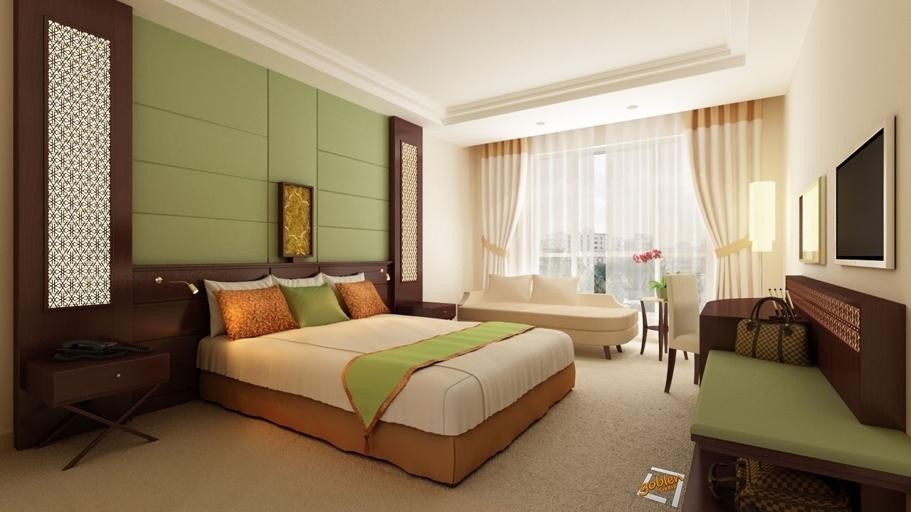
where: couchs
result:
[458,273,639,360]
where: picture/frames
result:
[795,175,827,265]
[278,181,314,259]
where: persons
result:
[594,257,606,293]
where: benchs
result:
[681,275,911,512]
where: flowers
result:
[632,248,661,264]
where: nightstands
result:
[397,302,456,319]
[19,345,171,472]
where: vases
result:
[655,258,665,297]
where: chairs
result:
[664,273,700,393]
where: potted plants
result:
[648,277,667,299]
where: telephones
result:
[54,340,124,361]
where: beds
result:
[132,261,575,486]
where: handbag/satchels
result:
[706,457,852,511]
[734,297,810,367]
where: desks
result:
[694,298,782,386]
[640,297,688,361]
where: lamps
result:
[748,180,775,298]
[379,268,391,281]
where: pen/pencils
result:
[770,288,795,317]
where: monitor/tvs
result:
[833,117,896,270]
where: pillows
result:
[155,276,199,295]
[203,274,273,338]
[271,272,324,287]
[322,271,365,316]
[532,273,580,305]
[279,282,351,329]
[485,274,532,303]
[212,285,301,341]
[335,279,390,319]
